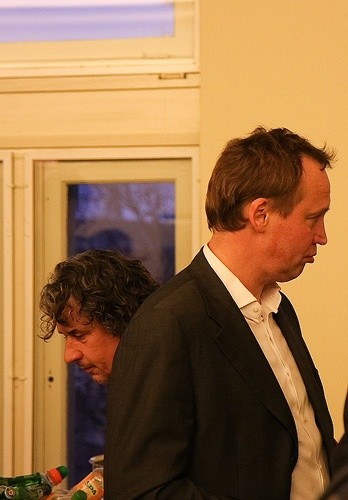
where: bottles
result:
[0,465,69,500]
[66,468,104,500]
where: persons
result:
[103,125,348,500]
[36,248,162,385]
